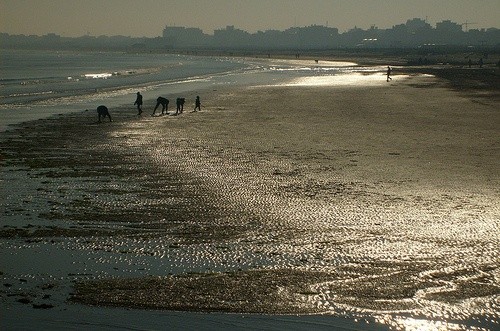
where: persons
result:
[151,97,169,116]
[479,56,483,68]
[194,96,201,111]
[469,56,473,68]
[176,98,185,116]
[134,91,143,115]
[97,105,113,124]
[387,66,392,82]
